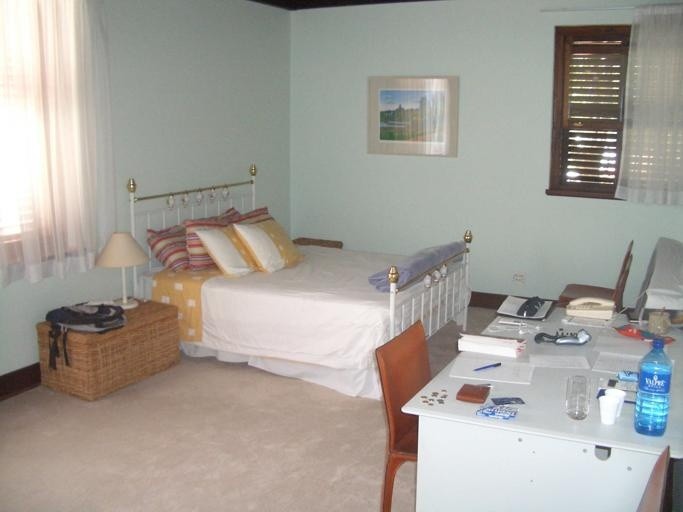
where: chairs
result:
[375,319,431,512]
[558,240,633,313]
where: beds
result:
[126,163,472,401]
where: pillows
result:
[147,205,305,281]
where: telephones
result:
[565,297,616,320]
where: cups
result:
[599,396,620,426]
[604,389,627,417]
[565,374,591,421]
[647,311,673,336]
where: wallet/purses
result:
[457,384,490,403]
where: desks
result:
[35,297,182,401]
[401,307,683,512]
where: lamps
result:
[94,232,149,311]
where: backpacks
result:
[46,302,127,369]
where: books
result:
[455,329,526,359]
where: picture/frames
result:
[367,76,459,157]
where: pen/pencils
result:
[472,362,503,372]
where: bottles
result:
[634,339,673,438]
